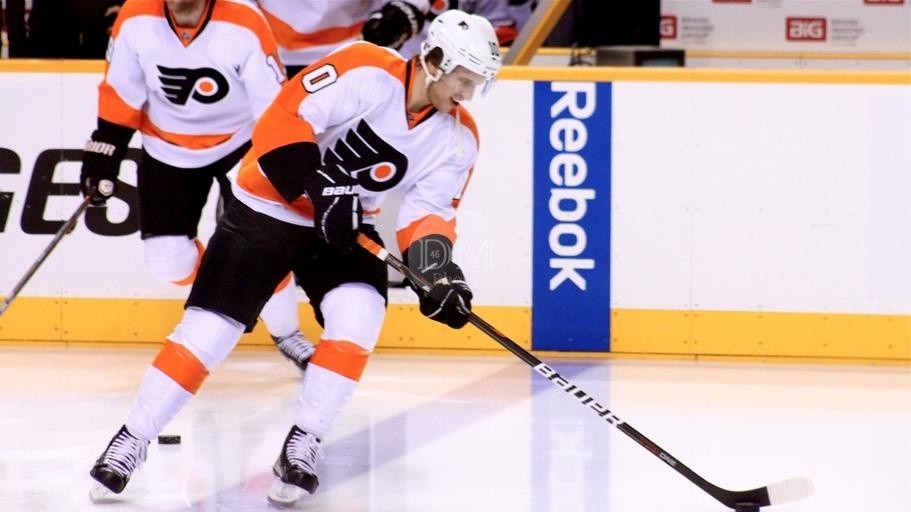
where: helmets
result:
[419,9,502,97]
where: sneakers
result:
[273,329,315,370]
[273,425,321,493]
[91,424,151,492]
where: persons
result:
[0,0,569,63]
[85,9,505,496]
[79,2,315,373]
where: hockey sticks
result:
[357,233,813,506]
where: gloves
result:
[403,236,472,329]
[361,2,424,51]
[80,130,126,205]
[305,164,363,244]
